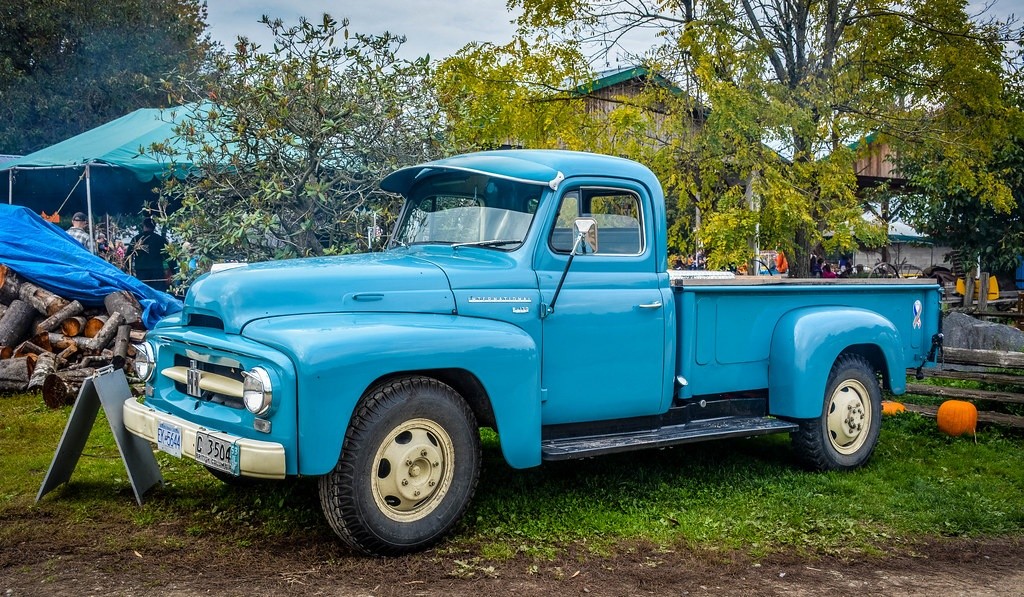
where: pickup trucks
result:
[118,149,947,560]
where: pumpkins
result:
[882,400,907,415]
[937,399,976,436]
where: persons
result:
[66,212,97,256]
[840,263,865,278]
[97,232,124,260]
[810,255,836,278]
[124,218,177,292]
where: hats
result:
[98,234,107,240]
[73,211,87,222]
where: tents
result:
[0,98,372,255]
